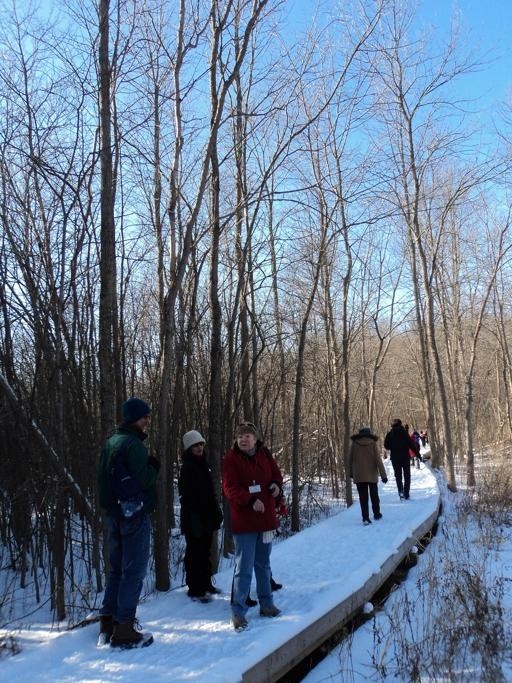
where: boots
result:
[97,613,113,644]
[109,618,153,649]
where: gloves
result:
[382,478,388,483]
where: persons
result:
[178,430,223,603]
[404,423,428,469]
[222,495,286,607]
[219,421,282,631]
[99,398,161,649]
[349,427,388,524]
[384,418,422,499]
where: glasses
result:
[239,422,256,431]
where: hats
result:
[359,428,370,434]
[120,396,152,424]
[182,429,207,451]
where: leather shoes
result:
[245,595,257,606]
[191,589,212,602]
[260,603,282,616]
[270,578,282,591]
[362,516,372,523]
[232,614,249,632]
[207,581,221,594]
[373,512,382,520]
